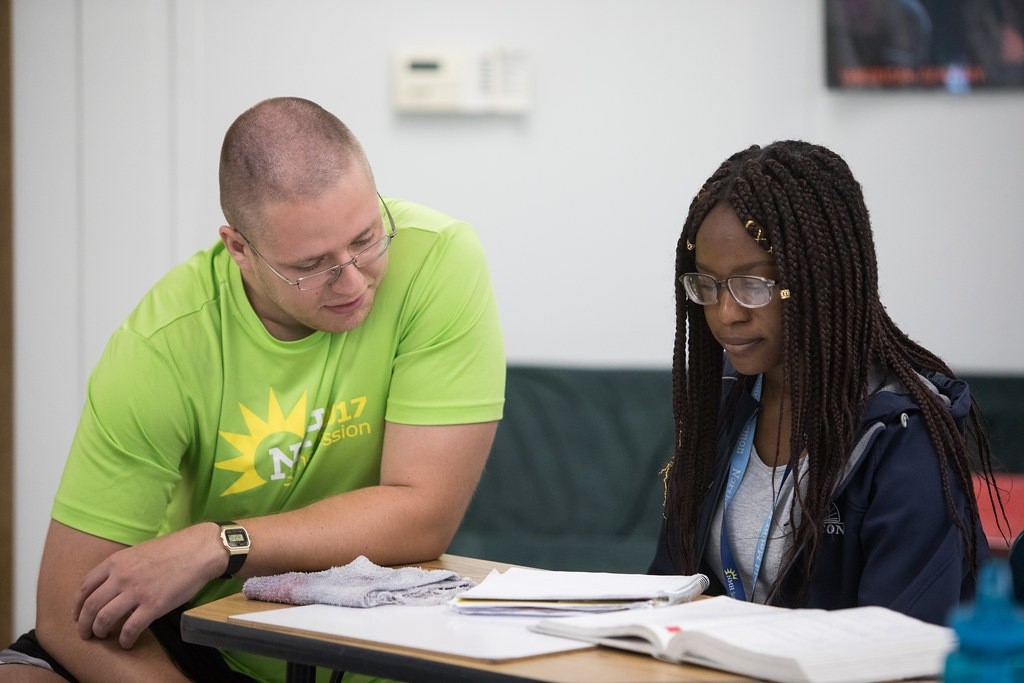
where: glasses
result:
[234,192,398,291]
[678,272,781,308]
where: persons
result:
[646,140,1012,627]
[0,96,508,683]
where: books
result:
[529,595,959,683]
[450,566,710,618]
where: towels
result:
[242,555,475,608]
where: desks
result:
[180,553,761,683]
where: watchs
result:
[213,520,252,580]
